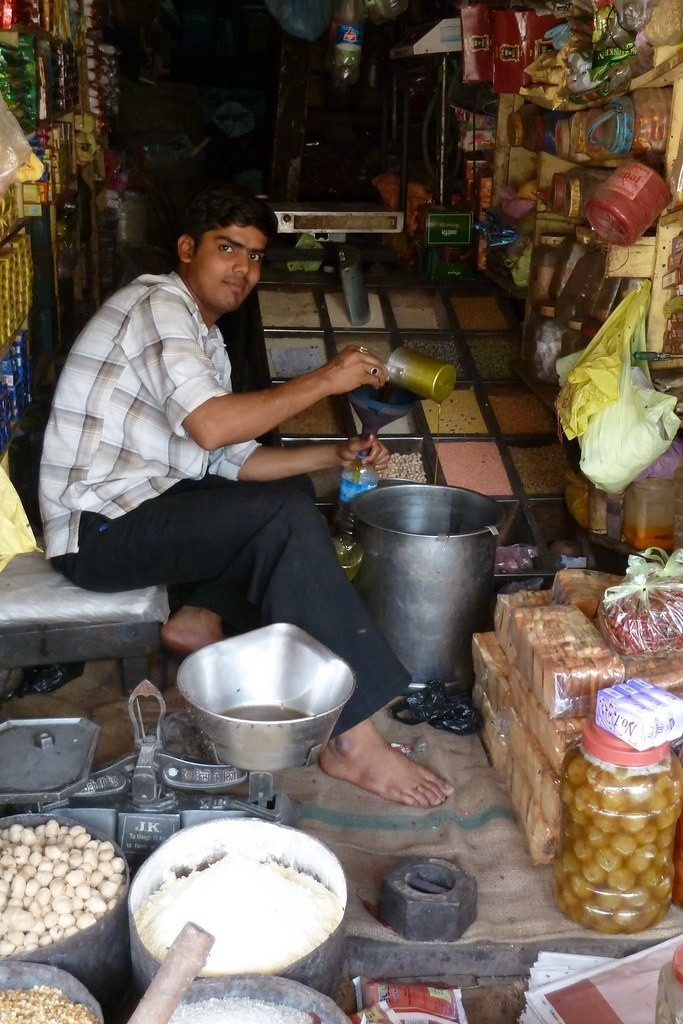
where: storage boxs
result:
[464,157,494,272]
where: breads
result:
[471,570,683,865]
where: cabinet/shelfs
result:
[494,0,683,559]
[0,217,38,466]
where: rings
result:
[359,347,363,352]
[371,365,378,376]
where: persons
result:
[37,189,456,808]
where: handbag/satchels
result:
[556,284,680,492]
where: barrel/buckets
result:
[351,481,505,686]
[1,811,355,1024]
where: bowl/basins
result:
[175,620,356,770]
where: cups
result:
[379,345,457,403]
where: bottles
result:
[655,942,683,1024]
[549,719,683,934]
[330,0,364,88]
[334,449,379,544]
[621,476,676,550]
[507,87,671,385]
[364,0,408,25]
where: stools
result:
[1,550,169,695]
